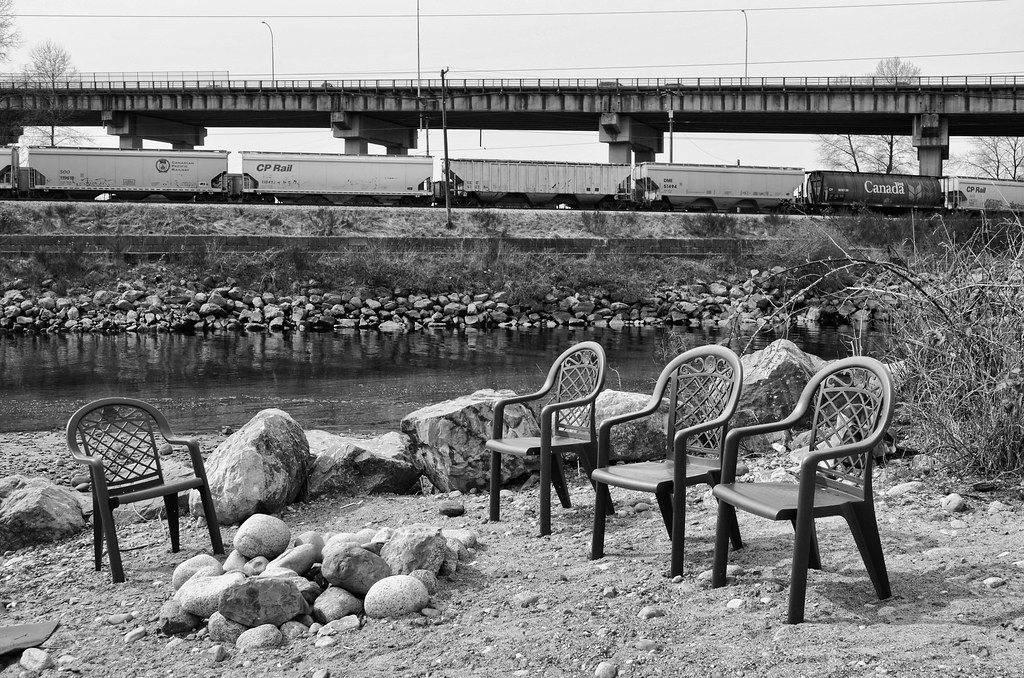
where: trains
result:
[0,143,1024,220]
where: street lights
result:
[741,10,748,84]
[260,20,275,87]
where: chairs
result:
[485,341,614,535]
[711,355,894,624]
[590,344,743,576]
[66,396,226,582]
